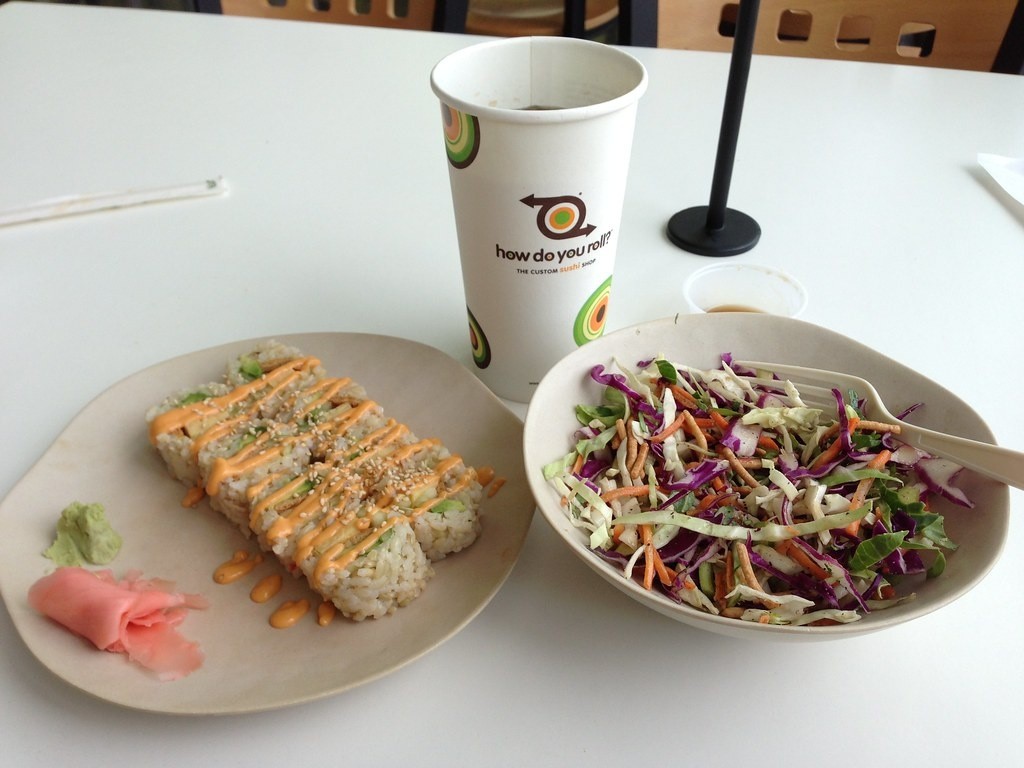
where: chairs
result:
[215,0,470,32]
[628,0,1019,75]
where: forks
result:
[732,361,1024,492]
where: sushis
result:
[146,338,483,621]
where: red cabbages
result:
[575,352,973,614]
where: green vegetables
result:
[543,362,957,623]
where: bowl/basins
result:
[523,312,1011,642]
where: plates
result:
[0,332,537,714]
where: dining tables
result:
[0,0,1024,768]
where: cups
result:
[682,261,807,315]
[429,35,648,404]
